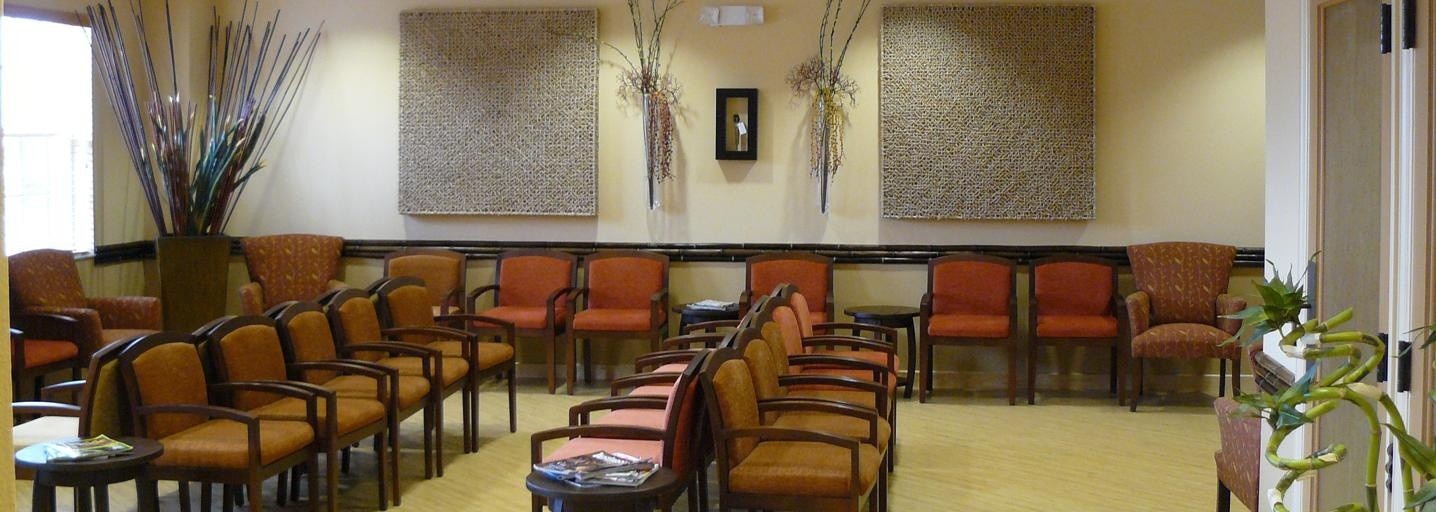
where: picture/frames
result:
[714,86,759,161]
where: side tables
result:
[843,307,925,400]
[526,455,681,511]
[15,439,165,509]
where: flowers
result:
[783,0,877,181]
[75,0,328,235]
[539,0,689,186]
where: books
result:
[533,449,660,490]
[39,433,134,463]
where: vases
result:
[156,223,230,336]
[813,92,837,218]
[640,93,660,212]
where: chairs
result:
[739,253,833,350]
[7,246,162,467]
[1126,242,1248,412]
[121,332,319,510]
[383,248,466,327]
[533,290,899,475]
[466,248,577,394]
[1027,255,1127,406]
[566,250,669,395]
[525,349,710,511]
[700,347,881,510]
[196,276,517,512]
[919,254,1016,404]
[1213,397,1260,507]
